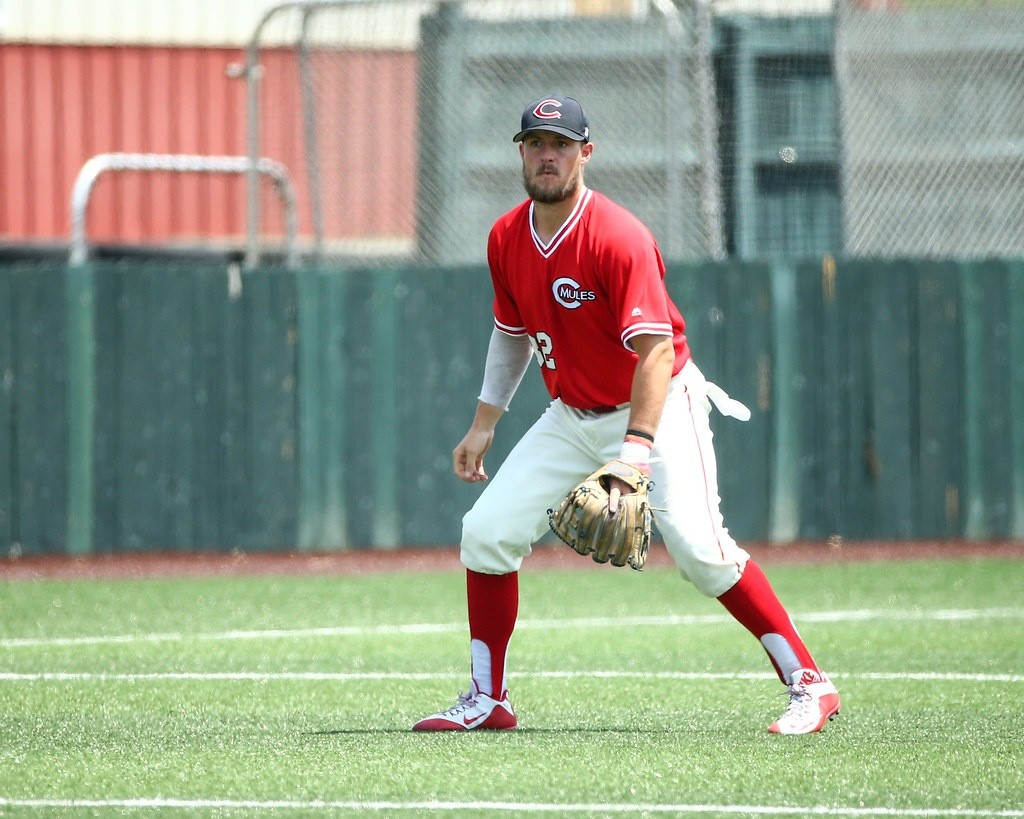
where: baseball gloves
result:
[548,459,655,570]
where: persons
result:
[412,96,840,736]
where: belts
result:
[585,405,615,413]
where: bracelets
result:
[621,442,650,465]
[626,429,654,443]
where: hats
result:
[513,95,590,143]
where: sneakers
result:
[768,670,840,736]
[412,689,517,733]
